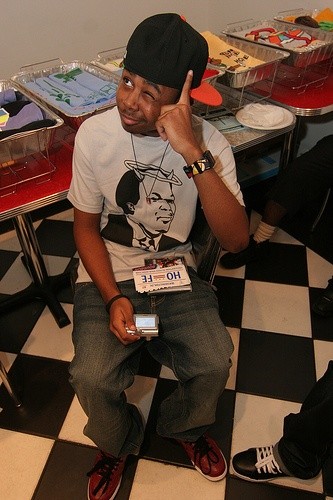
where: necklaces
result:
[130,132,169,202]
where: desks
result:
[0,82,296,328]
[248,58,333,117]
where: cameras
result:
[124,313,161,341]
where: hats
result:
[119,12,222,107]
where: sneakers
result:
[230,445,294,482]
[176,430,228,482]
[86,449,129,500]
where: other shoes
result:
[220,234,272,269]
[311,278,333,317]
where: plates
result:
[236,105,293,130]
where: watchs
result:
[182,149,216,179]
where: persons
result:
[68,13,248,500]
[230,361,333,482]
[220,134,333,317]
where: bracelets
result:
[106,293,128,314]
